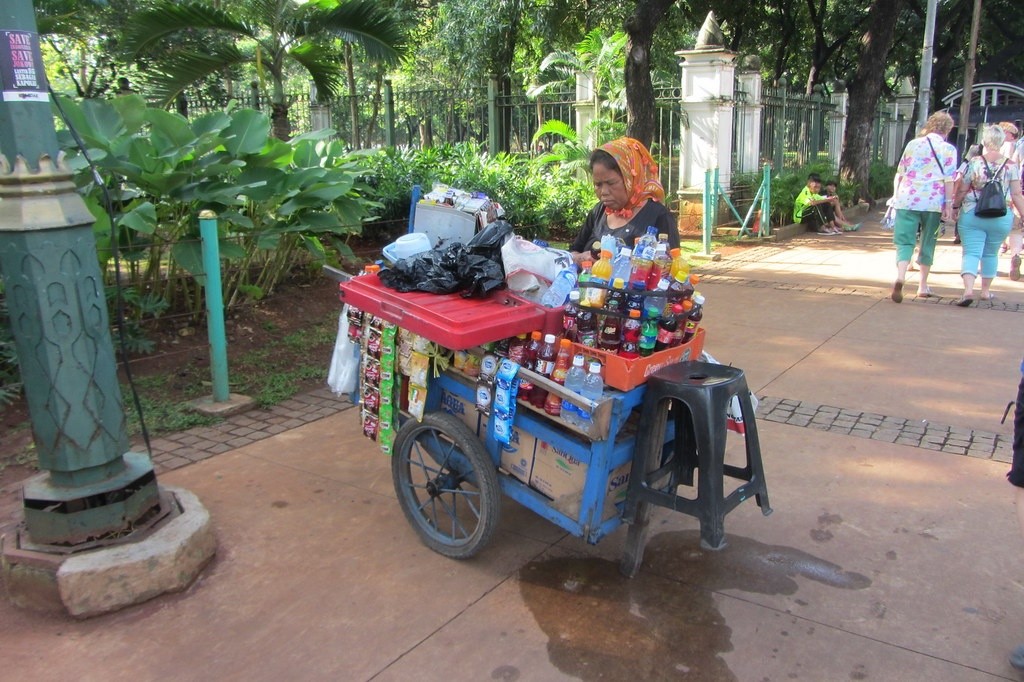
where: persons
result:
[792,173,863,236]
[889,113,958,303]
[952,124,1024,307]
[917,122,1024,280]
[569,137,679,265]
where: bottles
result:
[682,275,705,344]
[363,260,387,274]
[563,291,597,348]
[517,330,543,401]
[621,281,646,359]
[670,299,693,348]
[527,334,557,408]
[453,333,528,398]
[635,278,670,357]
[587,245,632,308]
[654,271,687,352]
[598,278,629,356]
[543,339,571,415]
[560,355,603,433]
[578,261,592,302]
[633,225,690,291]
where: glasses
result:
[1010,132,1018,139]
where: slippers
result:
[956,295,974,307]
[917,286,928,297]
[981,292,994,300]
[891,279,904,303]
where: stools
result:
[623,361,772,553]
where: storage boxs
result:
[439,389,479,452]
[339,274,567,352]
[530,438,672,506]
[544,328,706,394]
[478,413,537,487]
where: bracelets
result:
[953,206,959,210]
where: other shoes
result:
[914,245,920,254]
[1001,244,1007,253]
[907,263,913,270]
[954,234,960,244]
[818,222,862,233]
[1009,254,1021,281]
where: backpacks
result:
[972,155,1009,219]
